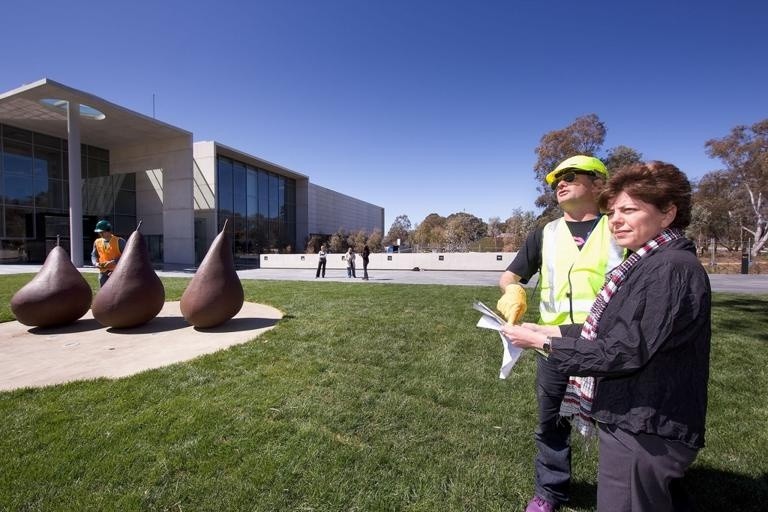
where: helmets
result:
[544,154,611,184]
[94,220,112,233]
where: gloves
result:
[492,283,528,325]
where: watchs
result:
[541,337,551,355]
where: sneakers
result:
[521,495,558,512]
[347,274,369,280]
[314,274,325,279]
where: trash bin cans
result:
[742,254,748,274]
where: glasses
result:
[551,171,593,191]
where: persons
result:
[358,245,369,280]
[314,246,326,279]
[343,247,356,279]
[494,153,631,512]
[500,159,711,512]
[89,219,126,287]
